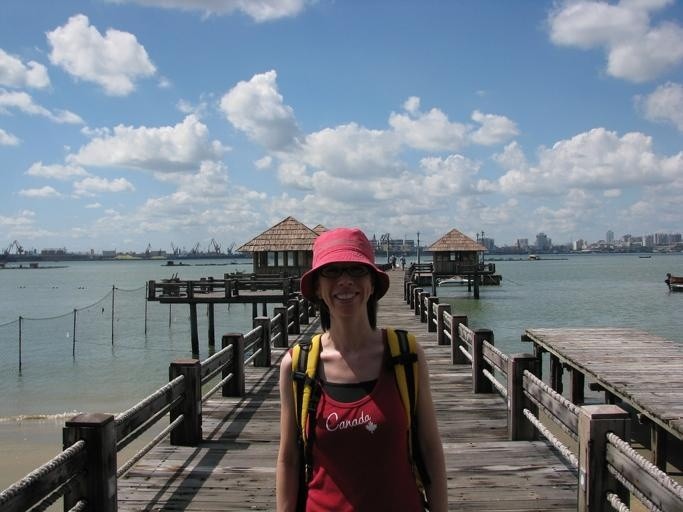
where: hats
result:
[299,228,390,303]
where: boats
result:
[665,272,683,291]
[161,261,253,266]
[0,262,68,270]
[639,256,651,258]
[438,276,475,287]
[485,255,568,261]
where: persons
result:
[389,253,397,271]
[400,255,406,270]
[276,227,450,512]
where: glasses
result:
[319,264,369,279]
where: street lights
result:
[377,233,390,263]
[476,230,484,271]
[416,230,420,270]
